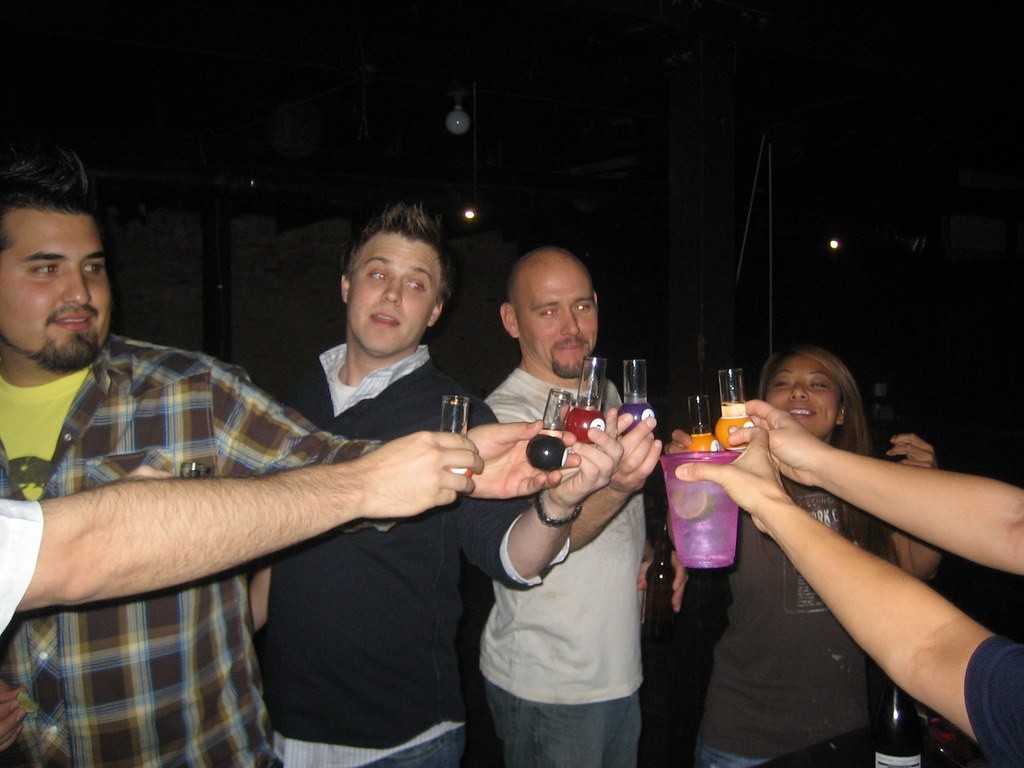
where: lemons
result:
[673,489,715,521]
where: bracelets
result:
[535,488,582,528]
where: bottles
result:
[876,685,928,768]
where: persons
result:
[479,246,688,768]
[0,138,1024,768]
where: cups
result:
[660,452,743,569]
